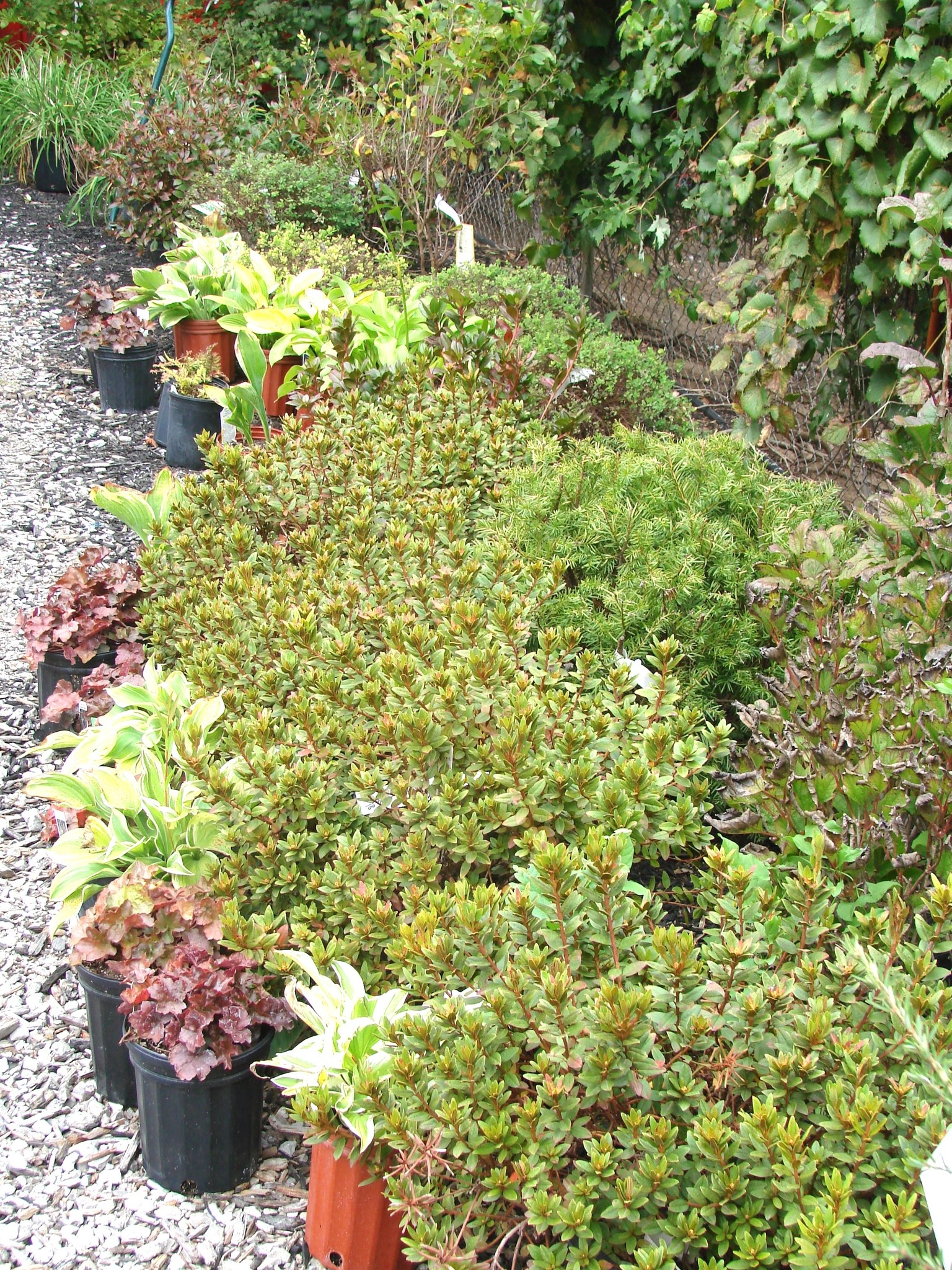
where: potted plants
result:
[18,213,952,1270]
